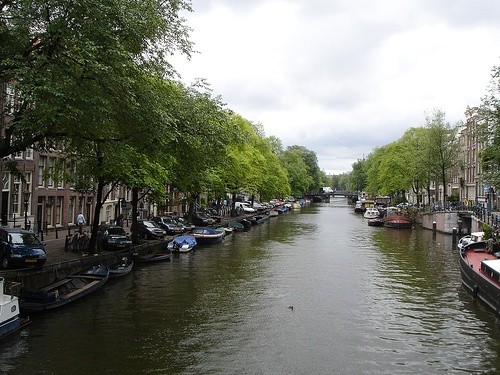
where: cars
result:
[130,214,195,239]
[97,225,132,251]
[0,228,47,270]
[319,187,333,193]
[235,199,284,214]
[396,202,441,213]
[183,211,215,226]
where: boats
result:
[137,248,172,264]
[18,263,109,315]
[458,232,500,317]
[265,199,305,217]
[354,201,412,229]
[107,257,134,278]
[0,278,32,340]
[167,222,244,254]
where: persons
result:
[77,213,87,230]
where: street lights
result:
[22,188,30,230]
[118,197,126,215]
